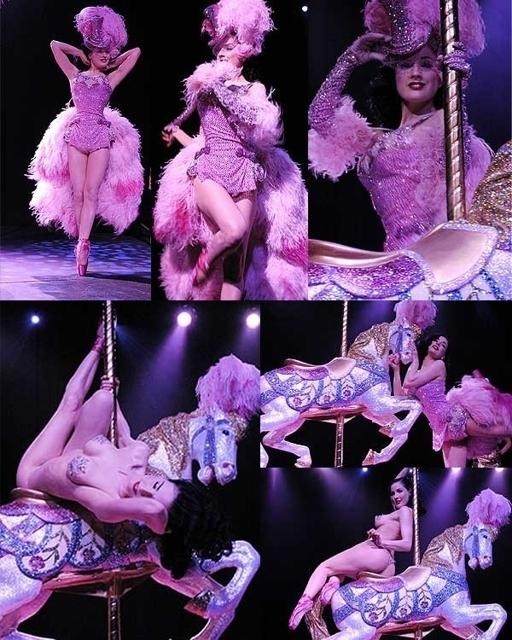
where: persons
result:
[47,38,143,276]
[162,32,271,300]
[388,333,512,468]
[309,34,493,255]
[287,468,427,631]
[14,316,234,562]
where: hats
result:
[203,4,234,56]
[84,16,113,51]
[378,0,432,64]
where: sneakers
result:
[74,239,90,276]
[192,247,214,286]
[320,575,341,606]
[288,594,314,631]
[92,316,117,354]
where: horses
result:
[260,313,424,468]
[0,389,262,640]
[316,514,510,640]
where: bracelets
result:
[381,541,384,547]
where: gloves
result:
[331,33,393,70]
[436,42,472,175]
[197,82,256,141]
